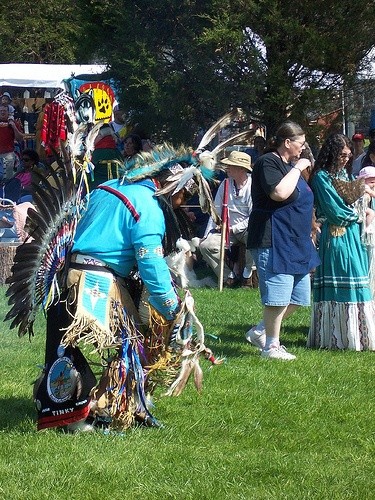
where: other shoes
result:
[240,271,253,289]
[223,272,239,289]
[56,420,93,436]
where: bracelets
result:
[294,167,302,173]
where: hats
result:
[352,134,364,141]
[220,150,253,173]
[357,166,375,180]
[0,92,12,101]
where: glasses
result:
[338,153,352,159]
[20,157,32,162]
[289,139,306,148]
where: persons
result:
[0,91,49,243]
[198,151,257,289]
[36,161,192,434]
[245,122,320,360]
[295,131,375,352]
[245,136,267,164]
[89,122,143,193]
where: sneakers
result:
[245,326,266,348]
[260,345,296,361]
